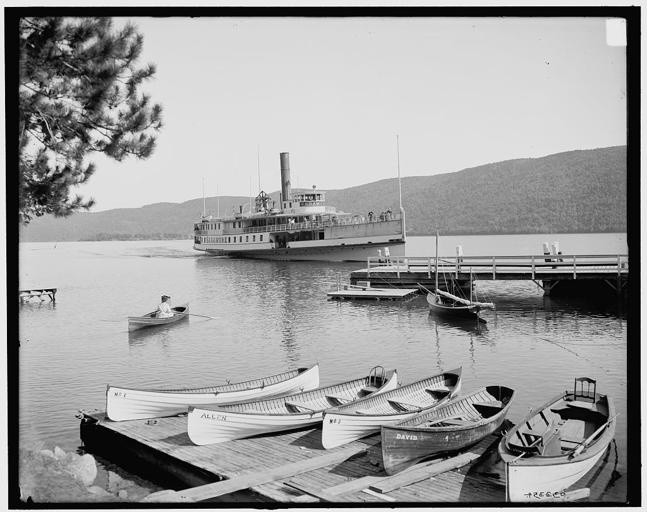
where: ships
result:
[192,135,406,262]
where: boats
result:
[326,276,419,302]
[496,376,616,504]
[108,364,318,420]
[128,305,189,326]
[417,229,493,314]
[189,365,397,445]
[321,364,463,450]
[380,383,514,476]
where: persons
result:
[156,296,175,318]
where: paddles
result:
[573,412,621,458]
[173,311,220,320]
[512,417,555,462]
[413,413,467,428]
